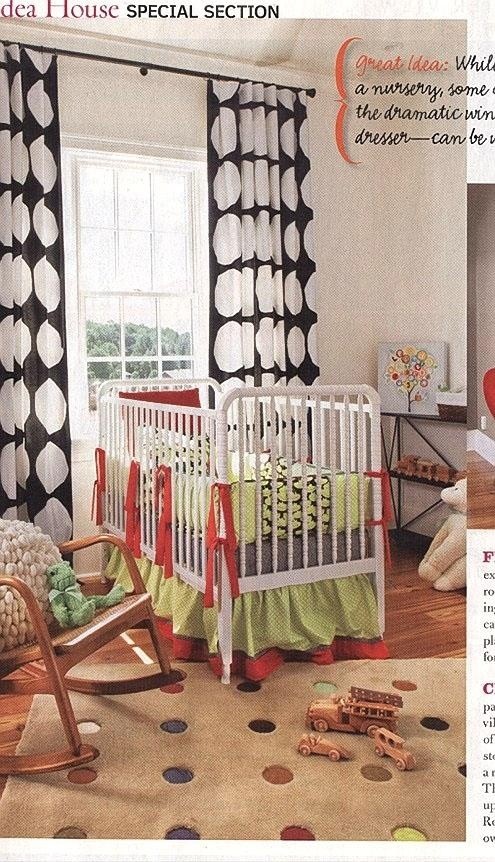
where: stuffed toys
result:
[304,685,404,738]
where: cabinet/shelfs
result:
[380,409,467,545]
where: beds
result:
[96,370,390,685]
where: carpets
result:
[0,655,466,840]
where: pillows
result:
[136,421,218,482]
[1,514,62,656]
[119,388,202,458]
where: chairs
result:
[0,514,187,779]
